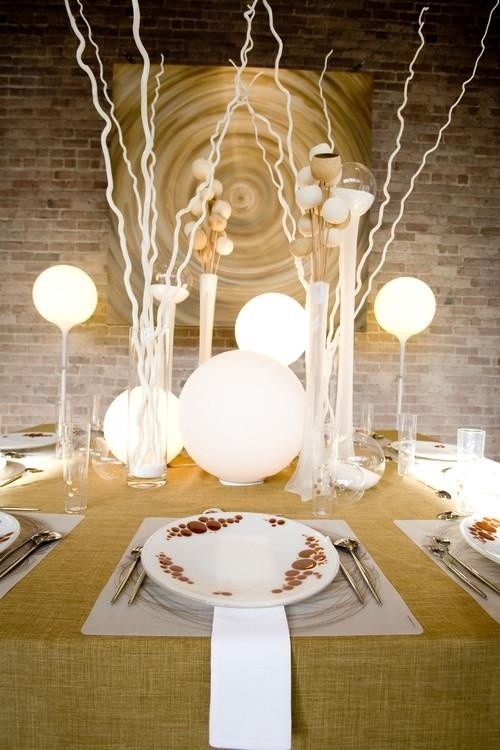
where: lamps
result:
[30,263,100,437]
[104,387,184,467]
[176,350,307,485]
[372,276,437,434]
[235,293,308,366]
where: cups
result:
[54,399,73,445]
[397,412,420,479]
[310,427,338,519]
[85,392,102,432]
[450,427,488,516]
[60,422,93,513]
[359,403,376,437]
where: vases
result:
[285,280,333,504]
[127,323,172,490]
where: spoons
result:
[333,534,386,607]
[0,528,63,579]
[399,471,452,500]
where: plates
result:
[0,510,22,556]
[0,431,58,452]
[389,438,460,462]
[140,511,342,609]
[458,512,500,565]
[0,459,27,481]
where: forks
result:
[425,533,500,597]
[423,543,489,601]
[109,543,144,604]
